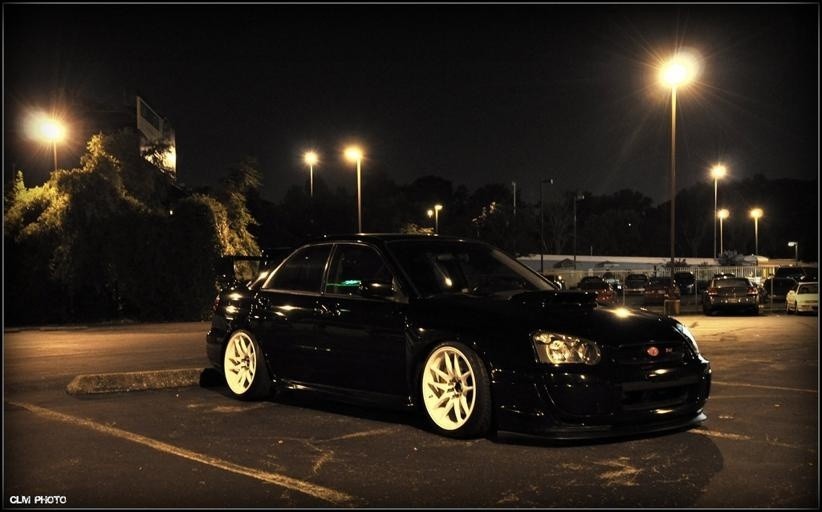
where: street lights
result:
[434,204,443,233]
[340,143,370,233]
[712,164,723,261]
[572,194,584,254]
[657,55,698,264]
[788,241,799,266]
[42,113,61,176]
[511,180,518,214]
[303,147,320,198]
[746,207,765,256]
[717,209,730,256]
[539,177,554,274]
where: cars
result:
[764,267,818,293]
[703,278,759,315]
[785,282,818,315]
[206,233,712,442]
